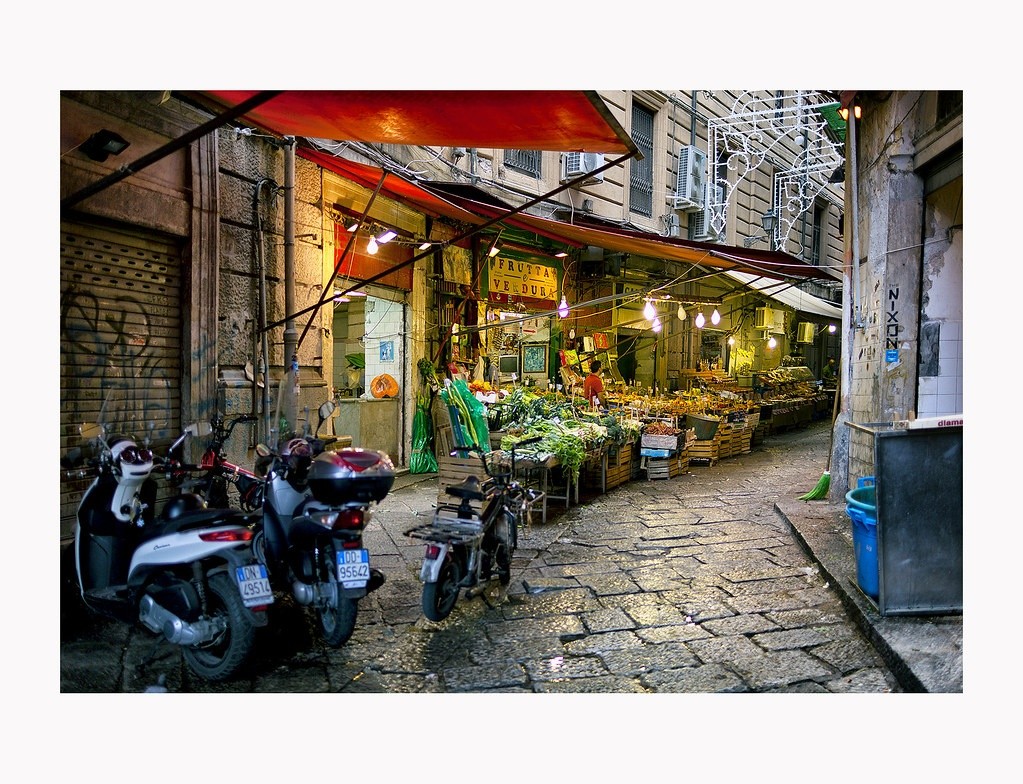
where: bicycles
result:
[195,412,267,511]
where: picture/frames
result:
[523,345,546,373]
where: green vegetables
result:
[484,386,606,484]
[416,357,440,386]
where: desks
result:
[493,445,607,524]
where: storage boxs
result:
[676,450,690,475]
[438,454,491,489]
[486,402,521,432]
[641,432,686,450]
[436,493,489,521]
[640,448,675,458]
[646,459,679,482]
[575,336,594,354]
[585,443,640,491]
[686,412,760,467]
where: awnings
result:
[418,183,844,367]
[295,144,505,369]
[715,267,843,329]
[60,90,644,334]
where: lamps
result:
[79,129,131,162]
[345,219,359,231]
[376,227,397,246]
[485,238,503,258]
[414,239,431,250]
[552,247,569,258]
[744,207,778,248]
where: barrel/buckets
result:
[842,474,880,598]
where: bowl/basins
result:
[684,413,723,441]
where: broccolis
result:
[589,415,623,437]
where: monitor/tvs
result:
[499,355,520,376]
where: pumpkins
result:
[370,373,399,399]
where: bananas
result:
[472,379,492,391]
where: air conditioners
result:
[694,182,723,241]
[797,322,815,343]
[567,152,604,182]
[755,307,775,330]
[676,144,708,210]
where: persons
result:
[584,359,609,411]
[820,358,837,386]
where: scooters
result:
[64,419,274,683]
[402,436,544,622]
[242,399,397,649]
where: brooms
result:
[798,364,842,501]
